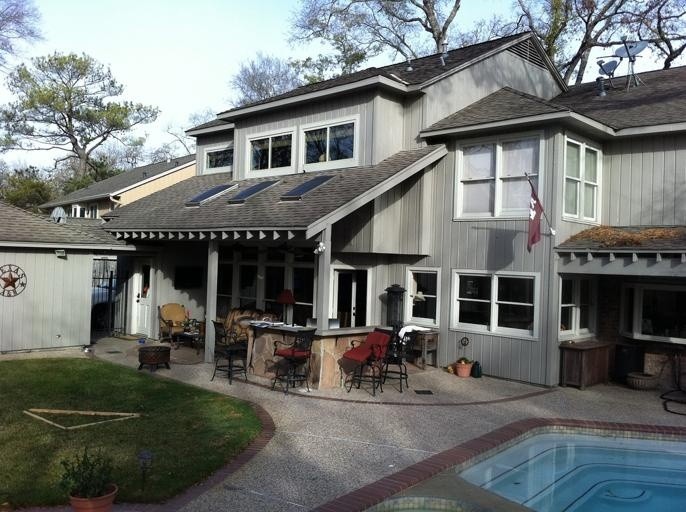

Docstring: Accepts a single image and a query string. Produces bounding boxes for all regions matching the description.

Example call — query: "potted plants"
[457,358,474,377]
[59,445,118,511]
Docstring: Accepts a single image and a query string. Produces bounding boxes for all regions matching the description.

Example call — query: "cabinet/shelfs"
[559,340,610,389]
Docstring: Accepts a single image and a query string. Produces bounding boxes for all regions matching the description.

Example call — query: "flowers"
[180,318,194,329]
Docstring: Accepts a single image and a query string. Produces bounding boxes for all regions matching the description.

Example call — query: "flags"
[525,190,544,254]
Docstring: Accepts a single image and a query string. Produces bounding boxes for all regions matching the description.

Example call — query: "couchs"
[223,308,278,358]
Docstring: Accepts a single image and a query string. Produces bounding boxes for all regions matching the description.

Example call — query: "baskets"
[627,370,658,390]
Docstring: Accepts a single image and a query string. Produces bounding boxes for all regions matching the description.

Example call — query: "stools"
[138,346,171,373]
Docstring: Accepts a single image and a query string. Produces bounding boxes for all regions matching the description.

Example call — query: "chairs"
[158,303,197,350]
[342,328,411,397]
[271,327,317,395]
[209,319,248,385]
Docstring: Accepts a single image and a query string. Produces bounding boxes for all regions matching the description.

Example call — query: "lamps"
[276,290,296,324]
